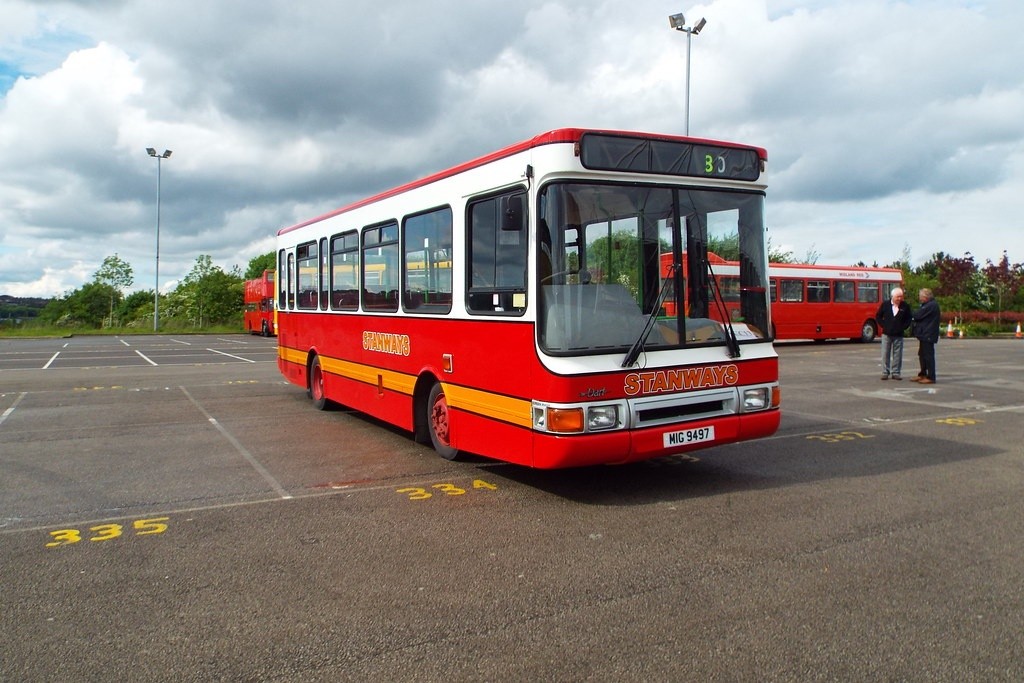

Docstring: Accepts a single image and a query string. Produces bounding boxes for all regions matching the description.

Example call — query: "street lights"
[146,147,173,332]
[667,12,707,140]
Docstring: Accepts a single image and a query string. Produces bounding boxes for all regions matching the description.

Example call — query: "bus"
[275,130,782,469]
[660,251,904,343]
[244,268,277,338]
[273,259,452,336]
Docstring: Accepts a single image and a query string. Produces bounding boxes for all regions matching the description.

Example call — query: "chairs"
[298,288,424,311]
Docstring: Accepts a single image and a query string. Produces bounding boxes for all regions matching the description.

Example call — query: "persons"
[912,288,941,384]
[876,287,912,380]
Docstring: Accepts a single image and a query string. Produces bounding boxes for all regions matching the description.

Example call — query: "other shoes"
[891,374,902,380]
[918,377,935,383]
[880,374,888,380]
[910,376,925,381]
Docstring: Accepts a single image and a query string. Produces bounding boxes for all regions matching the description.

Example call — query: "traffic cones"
[958,328,965,339]
[945,319,956,339]
[1014,320,1024,338]
